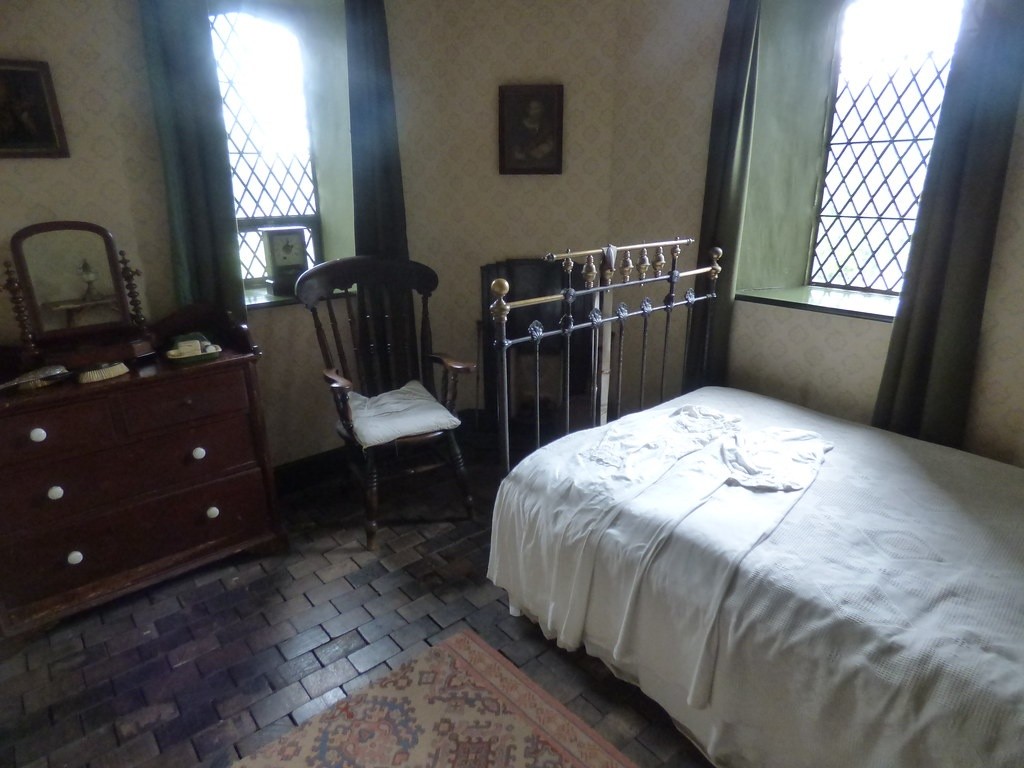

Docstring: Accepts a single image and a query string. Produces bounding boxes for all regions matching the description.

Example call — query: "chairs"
[294,256,474,551]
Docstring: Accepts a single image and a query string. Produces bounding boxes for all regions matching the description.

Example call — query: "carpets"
[228,628,639,768]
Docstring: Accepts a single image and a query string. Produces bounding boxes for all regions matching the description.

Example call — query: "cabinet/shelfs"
[0,309,290,642]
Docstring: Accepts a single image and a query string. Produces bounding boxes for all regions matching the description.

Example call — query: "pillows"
[349,379,462,450]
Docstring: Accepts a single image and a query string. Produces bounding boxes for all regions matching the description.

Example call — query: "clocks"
[260,227,308,296]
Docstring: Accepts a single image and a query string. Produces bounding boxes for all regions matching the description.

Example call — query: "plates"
[166,345,223,366]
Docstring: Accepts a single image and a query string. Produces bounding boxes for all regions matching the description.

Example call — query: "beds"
[488,236,1023,768]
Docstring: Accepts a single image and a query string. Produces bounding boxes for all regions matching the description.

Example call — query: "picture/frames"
[0,56,71,159]
[498,83,563,175]
